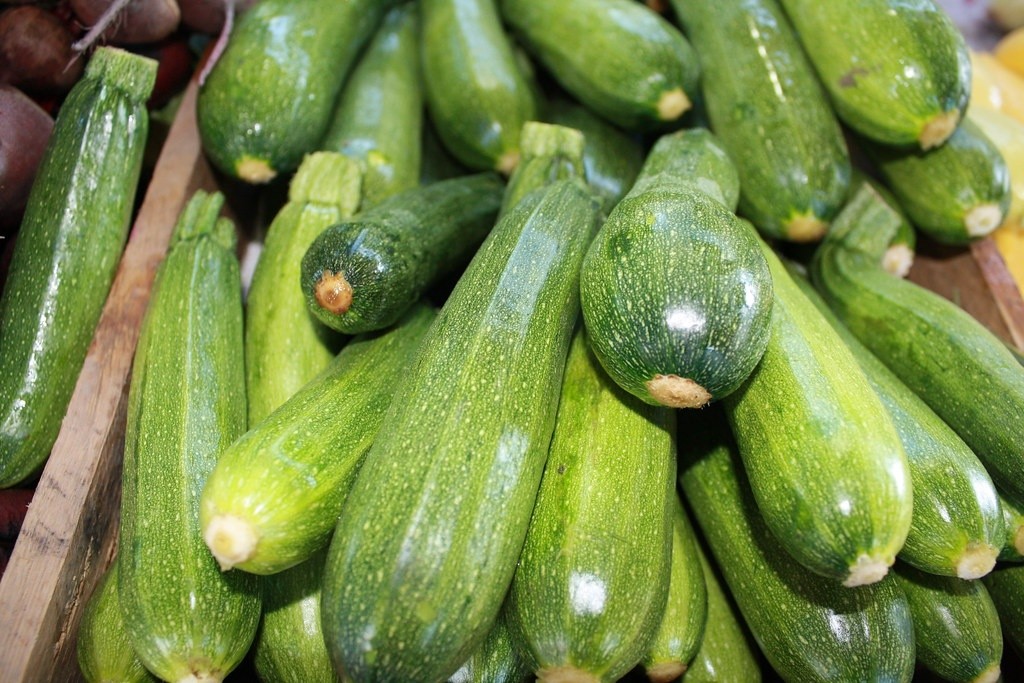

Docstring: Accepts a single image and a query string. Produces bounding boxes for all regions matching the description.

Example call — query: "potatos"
[0,0,252,227]
